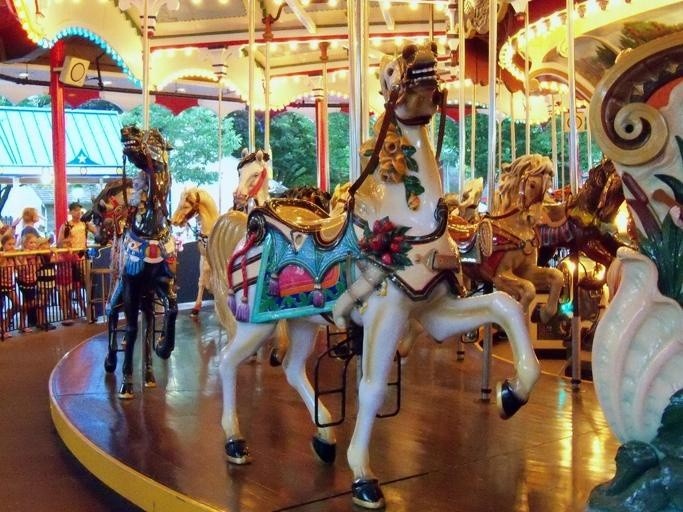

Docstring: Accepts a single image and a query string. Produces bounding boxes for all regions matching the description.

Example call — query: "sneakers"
[0,321,57,338]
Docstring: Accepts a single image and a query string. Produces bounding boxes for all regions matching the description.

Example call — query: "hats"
[70,203,83,210]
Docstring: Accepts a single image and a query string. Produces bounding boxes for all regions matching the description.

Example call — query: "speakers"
[59,56,90,88]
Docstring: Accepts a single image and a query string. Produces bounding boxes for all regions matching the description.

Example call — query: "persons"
[0,234,22,340]
[35,238,57,330]
[0,215,22,242]
[54,236,82,321]
[16,234,44,332]
[57,202,97,318]
[20,208,54,247]
